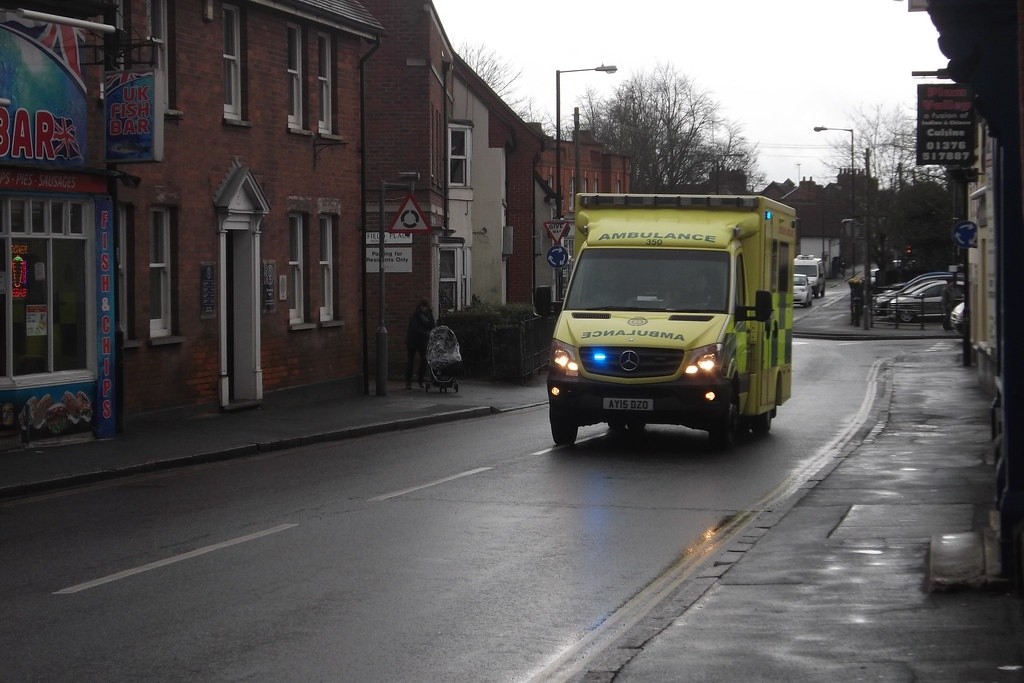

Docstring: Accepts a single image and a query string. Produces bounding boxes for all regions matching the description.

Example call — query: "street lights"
[556,65,617,299]
[814,127,855,278]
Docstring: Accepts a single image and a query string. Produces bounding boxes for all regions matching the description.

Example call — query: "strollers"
[423,326,463,393]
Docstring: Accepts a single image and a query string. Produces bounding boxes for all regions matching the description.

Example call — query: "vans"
[793,254,826,298]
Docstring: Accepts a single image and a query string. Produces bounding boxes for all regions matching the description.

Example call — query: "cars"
[793,273,812,307]
[871,268,967,323]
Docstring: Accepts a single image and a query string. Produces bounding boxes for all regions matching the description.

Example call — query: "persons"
[679,268,729,313]
[941,275,959,331]
[405,296,436,390]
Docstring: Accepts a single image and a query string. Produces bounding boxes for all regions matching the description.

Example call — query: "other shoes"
[417,379,425,387]
[406,383,411,389]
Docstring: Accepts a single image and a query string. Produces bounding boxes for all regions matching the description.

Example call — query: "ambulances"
[547,193,795,444]
